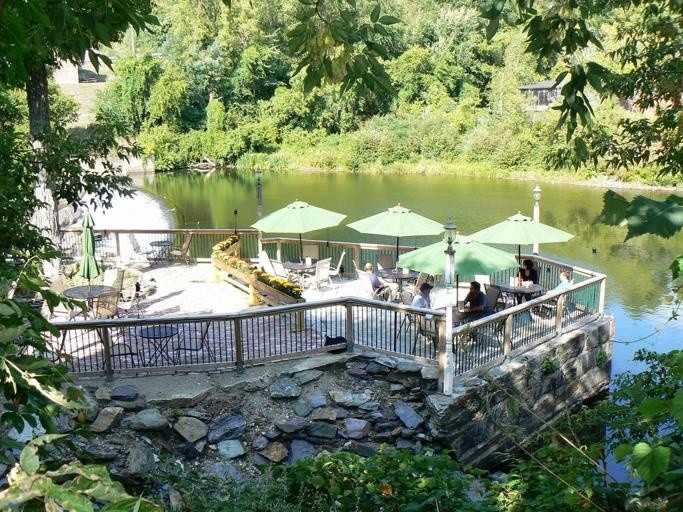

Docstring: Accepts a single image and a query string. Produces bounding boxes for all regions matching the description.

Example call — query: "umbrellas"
[345,202,444,264]
[395,231,518,305]
[466,212,575,269]
[250,197,348,262]
[77,206,101,289]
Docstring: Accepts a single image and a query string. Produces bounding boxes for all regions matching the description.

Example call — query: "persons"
[408,283,433,321]
[544,271,570,292]
[456,281,492,341]
[365,263,391,301]
[517,260,537,305]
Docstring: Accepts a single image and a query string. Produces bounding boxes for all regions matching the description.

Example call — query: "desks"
[139,326,177,367]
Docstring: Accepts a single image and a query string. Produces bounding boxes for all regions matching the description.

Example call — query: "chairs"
[97,328,144,369]
[259,250,346,294]
[176,310,213,365]
[353,255,541,357]
[127,233,197,269]
[48,269,139,321]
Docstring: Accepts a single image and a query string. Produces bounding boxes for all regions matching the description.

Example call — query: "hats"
[419,283,434,292]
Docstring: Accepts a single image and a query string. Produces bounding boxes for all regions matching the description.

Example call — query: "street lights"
[254,165,264,258]
[531,182,542,254]
[439,217,458,396]
